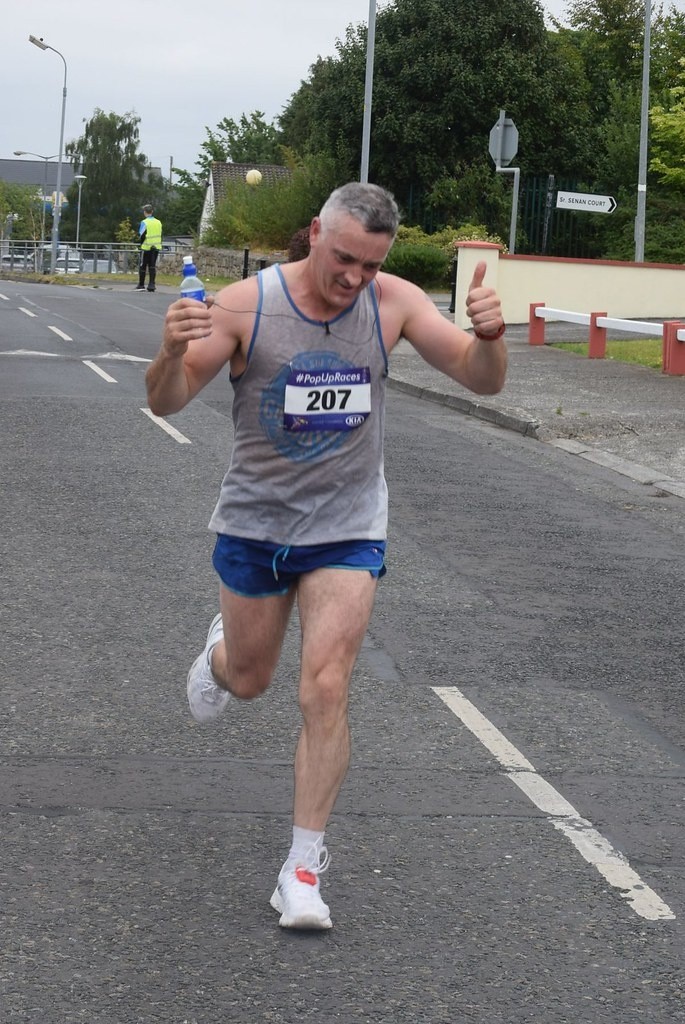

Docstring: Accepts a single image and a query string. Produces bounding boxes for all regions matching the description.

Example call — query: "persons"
[135,205,162,292]
[144,183,507,930]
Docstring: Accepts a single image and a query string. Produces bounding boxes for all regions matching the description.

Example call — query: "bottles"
[179,256,209,339]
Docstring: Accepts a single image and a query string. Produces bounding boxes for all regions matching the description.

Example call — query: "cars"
[30,245,83,271]
[81,259,117,275]
[1,255,34,272]
[54,257,84,273]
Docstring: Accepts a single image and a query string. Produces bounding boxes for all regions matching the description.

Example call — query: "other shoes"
[148,288,155,292]
[132,285,146,291]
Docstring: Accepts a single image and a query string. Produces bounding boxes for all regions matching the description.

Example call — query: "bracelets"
[474,323,505,340]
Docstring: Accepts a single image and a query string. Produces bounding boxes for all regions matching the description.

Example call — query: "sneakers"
[187,610,232,725]
[270,834,332,930]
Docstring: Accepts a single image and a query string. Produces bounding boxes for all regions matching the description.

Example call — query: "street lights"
[13,151,79,273]
[75,175,87,248]
[29,35,67,275]
